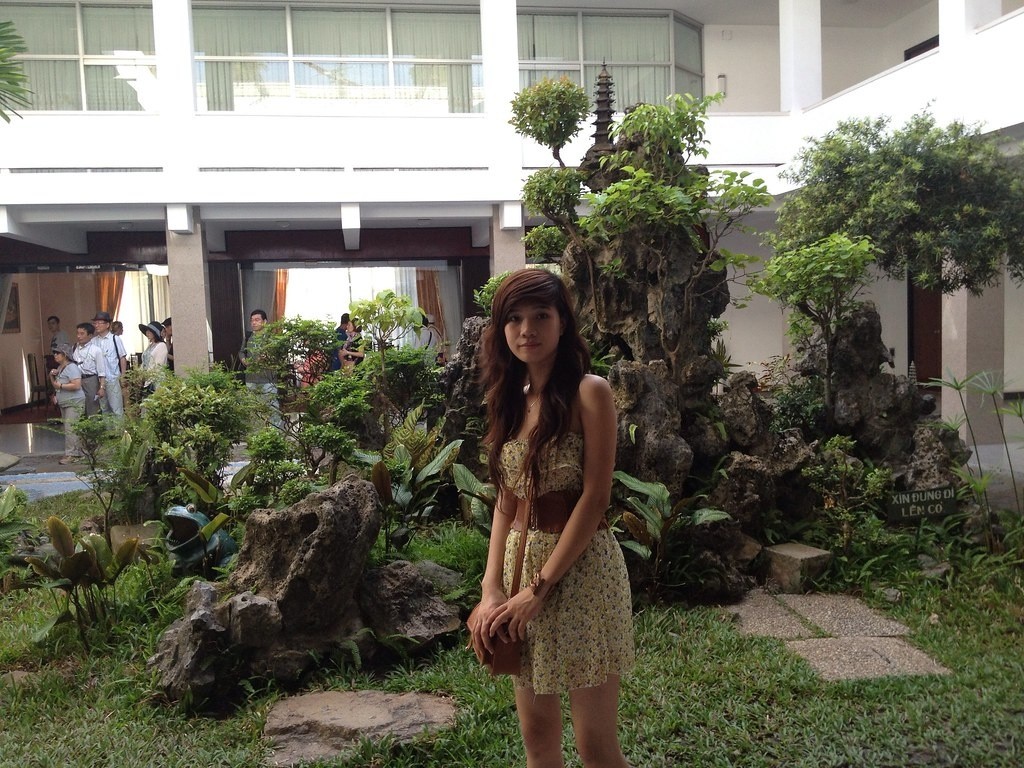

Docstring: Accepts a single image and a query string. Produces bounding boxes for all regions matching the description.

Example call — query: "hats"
[51,343,79,367]
[91,312,113,322]
[426,313,435,323]
[138,321,167,344]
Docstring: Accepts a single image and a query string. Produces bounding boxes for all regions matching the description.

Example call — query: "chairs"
[27,352,48,412]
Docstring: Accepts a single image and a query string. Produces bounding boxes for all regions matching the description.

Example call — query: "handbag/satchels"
[233,330,253,380]
[343,359,354,367]
[51,393,57,406]
[118,361,131,373]
[466,602,522,678]
[46,401,61,425]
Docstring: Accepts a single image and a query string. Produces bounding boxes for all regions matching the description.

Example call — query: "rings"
[51,371,53,372]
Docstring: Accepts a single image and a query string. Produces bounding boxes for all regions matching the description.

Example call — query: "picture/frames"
[2,283,21,334]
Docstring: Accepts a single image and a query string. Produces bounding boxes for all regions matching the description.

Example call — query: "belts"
[513,487,610,534]
[81,373,95,378]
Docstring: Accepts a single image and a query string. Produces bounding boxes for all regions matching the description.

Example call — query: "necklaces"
[527,396,540,413]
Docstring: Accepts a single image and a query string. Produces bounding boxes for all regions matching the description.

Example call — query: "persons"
[333,313,365,371]
[239,309,282,430]
[470,268,632,768]
[46,311,174,465]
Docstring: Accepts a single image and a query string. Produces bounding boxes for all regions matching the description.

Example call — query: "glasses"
[249,318,262,322]
[93,321,107,324]
[53,352,61,355]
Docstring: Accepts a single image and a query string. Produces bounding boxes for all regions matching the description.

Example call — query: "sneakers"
[58,456,77,464]
[78,455,86,461]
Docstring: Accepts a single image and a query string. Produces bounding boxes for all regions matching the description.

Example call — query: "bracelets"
[60,383,62,389]
[530,573,557,600]
[100,386,106,390]
